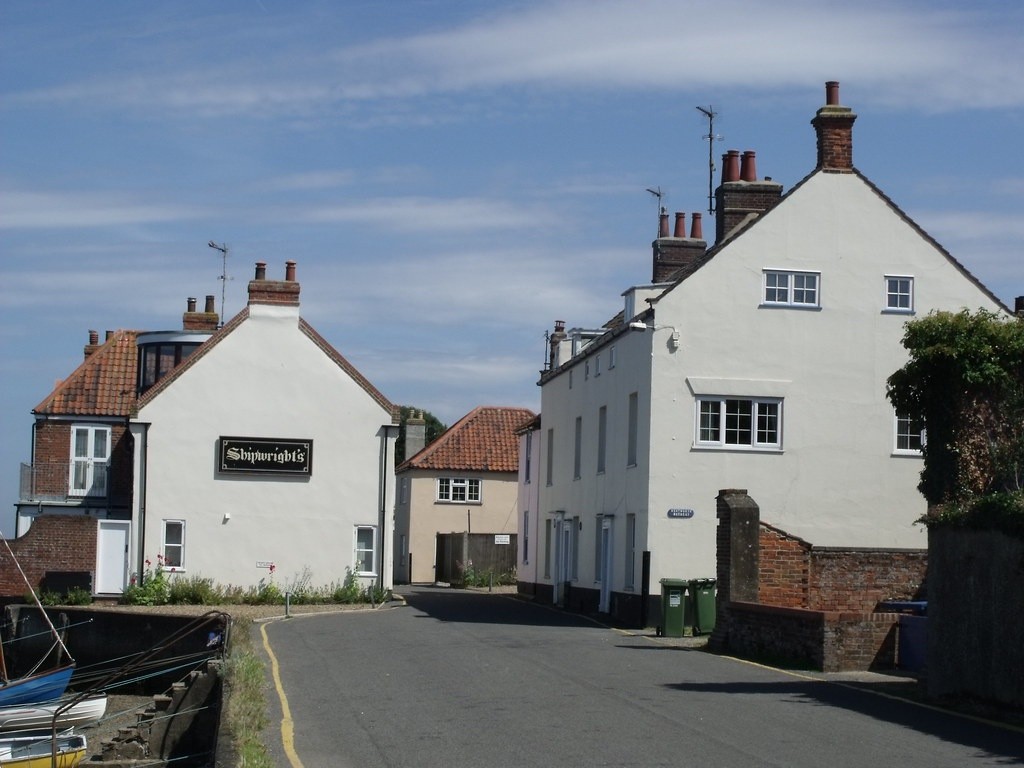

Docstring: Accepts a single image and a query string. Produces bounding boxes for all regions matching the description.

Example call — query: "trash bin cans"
[656,578,689,637]
[687,578,717,636]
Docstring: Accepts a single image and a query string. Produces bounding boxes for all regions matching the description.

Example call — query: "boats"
[0,733,87,768]
[0,725,74,736]
[1,530,76,705]
[0,691,108,730]
